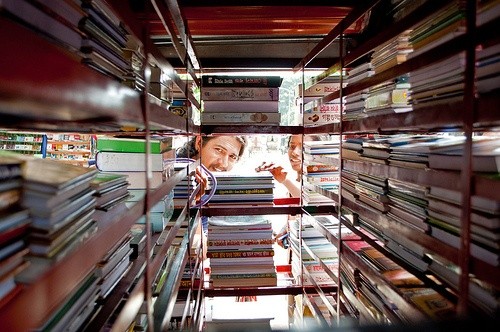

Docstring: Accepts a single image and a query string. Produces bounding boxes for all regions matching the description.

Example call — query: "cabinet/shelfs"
[0,1,500,331]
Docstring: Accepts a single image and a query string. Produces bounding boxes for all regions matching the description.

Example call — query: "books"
[203,174,275,206]
[200,72,284,125]
[289,0,499,331]
[174,173,341,332]
[0,0,204,332]
[208,215,277,287]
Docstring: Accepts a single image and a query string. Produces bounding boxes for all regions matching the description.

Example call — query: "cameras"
[279,234,289,250]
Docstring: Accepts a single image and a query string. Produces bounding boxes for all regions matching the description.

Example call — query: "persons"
[255,134,332,332]
[176,133,257,302]
